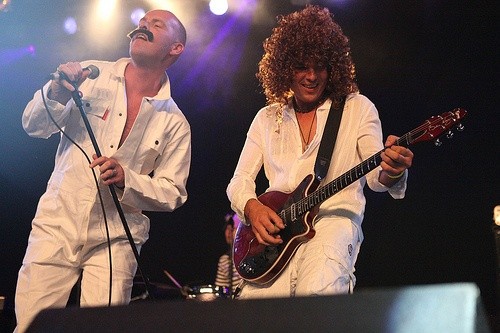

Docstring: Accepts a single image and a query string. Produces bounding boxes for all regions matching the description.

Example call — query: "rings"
[112,169,116,176]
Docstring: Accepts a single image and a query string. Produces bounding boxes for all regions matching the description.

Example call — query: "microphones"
[47,64,100,80]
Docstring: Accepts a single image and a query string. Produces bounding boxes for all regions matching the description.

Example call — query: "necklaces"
[293,96,317,151]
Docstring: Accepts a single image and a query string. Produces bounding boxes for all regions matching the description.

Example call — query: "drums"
[191,284,228,303]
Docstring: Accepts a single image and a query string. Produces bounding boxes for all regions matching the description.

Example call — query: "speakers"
[24,281,492,333]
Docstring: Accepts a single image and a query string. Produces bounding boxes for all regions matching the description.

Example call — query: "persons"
[226,5,412,298]
[215,216,241,293]
[13,9,192,333]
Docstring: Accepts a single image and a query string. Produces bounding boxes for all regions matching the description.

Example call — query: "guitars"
[233,108,467,289]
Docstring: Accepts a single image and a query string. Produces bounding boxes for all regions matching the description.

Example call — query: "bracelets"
[385,171,404,178]
